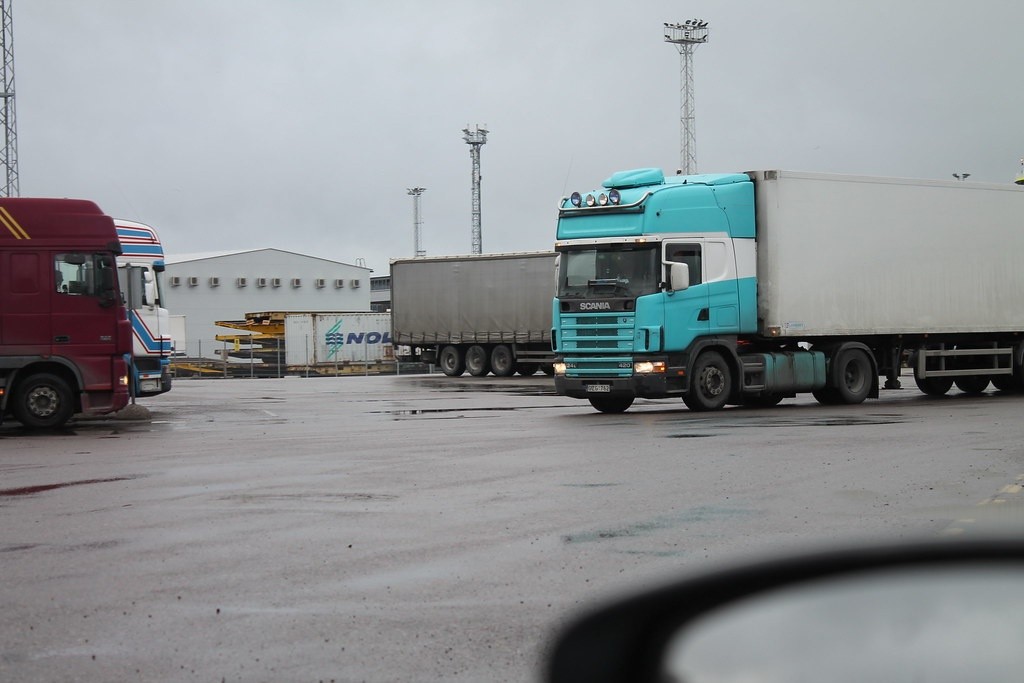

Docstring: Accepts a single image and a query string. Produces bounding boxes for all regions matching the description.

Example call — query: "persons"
[55,271,64,293]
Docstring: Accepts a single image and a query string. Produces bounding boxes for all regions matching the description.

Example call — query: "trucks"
[0,199,136,430]
[112,218,171,398]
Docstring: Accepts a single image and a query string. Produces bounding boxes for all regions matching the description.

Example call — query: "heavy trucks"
[391,255,560,375]
[546,173,1024,410]
